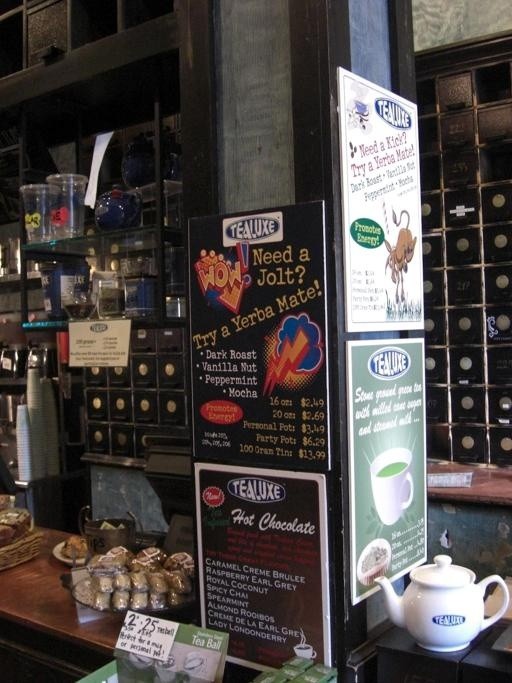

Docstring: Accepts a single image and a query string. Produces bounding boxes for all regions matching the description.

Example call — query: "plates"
[53,539,89,565]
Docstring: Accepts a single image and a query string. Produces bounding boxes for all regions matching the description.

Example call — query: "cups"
[46,173,89,240]
[294,644,317,660]
[19,183,61,245]
[370,447,415,525]
[155,656,176,683]
[16,369,60,481]
[63,288,97,321]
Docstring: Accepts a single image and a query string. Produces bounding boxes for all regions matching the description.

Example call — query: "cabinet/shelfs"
[0,0,220,459]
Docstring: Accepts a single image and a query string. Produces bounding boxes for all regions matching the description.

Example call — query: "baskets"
[0,529,45,570]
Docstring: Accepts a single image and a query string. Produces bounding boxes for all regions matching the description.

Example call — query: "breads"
[62,534,194,612]
[0,494,31,547]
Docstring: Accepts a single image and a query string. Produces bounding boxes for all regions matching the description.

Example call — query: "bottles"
[93,184,143,232]
[124,274,157,321]
[93,272,123,320]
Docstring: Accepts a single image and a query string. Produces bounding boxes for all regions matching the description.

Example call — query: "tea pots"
[373,554,510,654]
